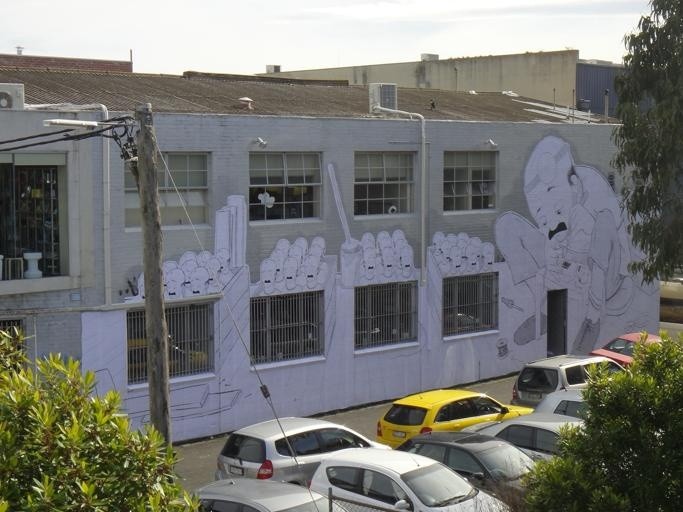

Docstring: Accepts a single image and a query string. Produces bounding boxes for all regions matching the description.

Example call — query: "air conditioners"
[0,83,24,111]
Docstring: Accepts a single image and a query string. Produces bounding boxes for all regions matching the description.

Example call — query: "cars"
[189,477,349,512]
[532,388,592,421]
[461,412,587,461]
[214,416,394,490]
[376,389,535,450]
[394,430,541,507]
[589,332,663,369]
[309,447,511,512]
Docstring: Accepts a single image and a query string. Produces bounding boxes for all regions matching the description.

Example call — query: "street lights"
[43,103,171,456]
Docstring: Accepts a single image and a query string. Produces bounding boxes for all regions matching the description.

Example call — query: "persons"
[492,135,634,357]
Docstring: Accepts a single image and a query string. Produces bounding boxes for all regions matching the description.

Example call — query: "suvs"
[510,354,632,409]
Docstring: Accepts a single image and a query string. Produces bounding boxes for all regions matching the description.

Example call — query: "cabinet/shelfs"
[18,168,61,276]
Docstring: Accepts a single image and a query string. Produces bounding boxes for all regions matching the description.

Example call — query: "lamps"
[483,138,498,148]
[254,136,267,147]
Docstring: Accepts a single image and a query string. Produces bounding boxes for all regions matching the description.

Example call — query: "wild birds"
[428,97,435,112]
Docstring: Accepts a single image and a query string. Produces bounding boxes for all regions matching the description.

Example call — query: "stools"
[5,257,24,280]
